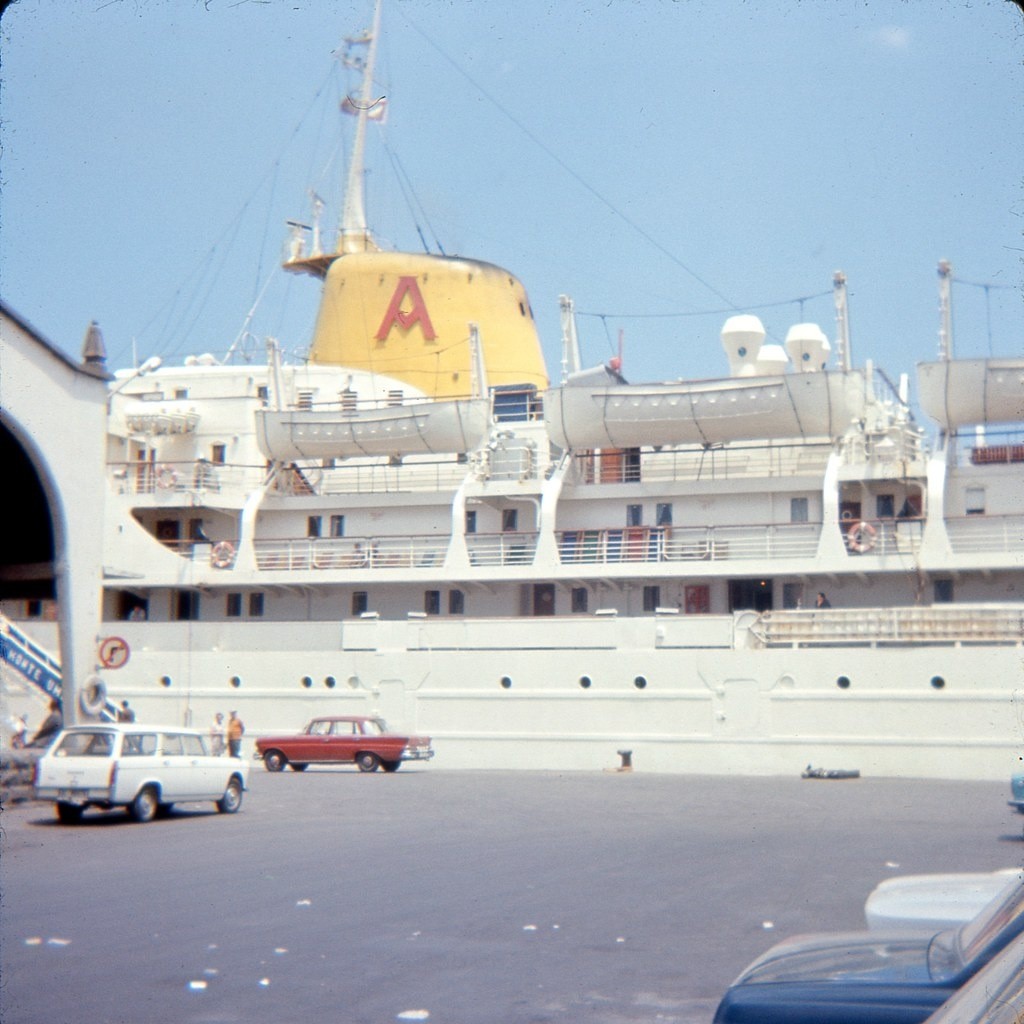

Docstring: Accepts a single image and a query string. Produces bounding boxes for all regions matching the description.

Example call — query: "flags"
[341,98,388,122]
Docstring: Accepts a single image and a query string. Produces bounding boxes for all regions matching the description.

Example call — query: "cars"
[256,715,435,775]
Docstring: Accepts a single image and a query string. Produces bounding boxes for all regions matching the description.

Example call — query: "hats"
[229,709,237,713]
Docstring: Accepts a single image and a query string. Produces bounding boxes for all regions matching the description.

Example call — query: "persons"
[128,605,146,621]
[119,701,135,723]
[815,593,831,609]
[211,709,245,756]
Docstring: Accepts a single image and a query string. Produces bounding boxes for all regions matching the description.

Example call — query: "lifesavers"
[210,540,235,568]
[153,464,178,488]
[849,521,877,553]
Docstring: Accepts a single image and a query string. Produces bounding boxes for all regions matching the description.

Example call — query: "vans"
[31,723,249,825]
